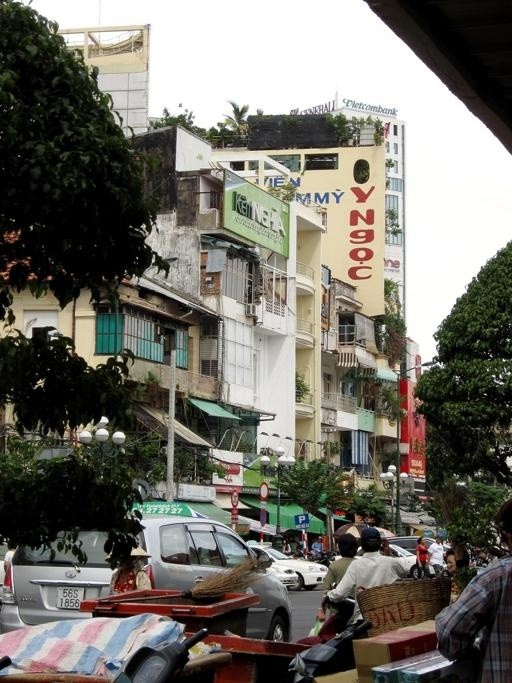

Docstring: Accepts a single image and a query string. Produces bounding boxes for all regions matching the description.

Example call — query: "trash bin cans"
[79,590,260,638]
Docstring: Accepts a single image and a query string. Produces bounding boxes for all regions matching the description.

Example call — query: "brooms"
[98,558,265,615]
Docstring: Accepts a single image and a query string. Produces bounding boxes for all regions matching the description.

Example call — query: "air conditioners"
[247,303,257,316]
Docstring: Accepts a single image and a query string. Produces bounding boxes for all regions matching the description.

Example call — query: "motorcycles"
[307,582,355,641]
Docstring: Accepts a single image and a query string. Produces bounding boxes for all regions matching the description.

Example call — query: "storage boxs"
[305,619,458,683]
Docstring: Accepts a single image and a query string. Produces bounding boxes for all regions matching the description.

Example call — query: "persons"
[424,534,445,578]
[315,532,359,622]
[311,536,324,552]
[321,526,411,627]
[281,537,292,553]
[435,499,512,683]
[415,536,427,578]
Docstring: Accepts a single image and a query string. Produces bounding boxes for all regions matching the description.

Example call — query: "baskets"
[355,569,452,639]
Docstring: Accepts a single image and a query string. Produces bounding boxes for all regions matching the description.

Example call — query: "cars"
[248,544,329,591]
[330,536,476,580]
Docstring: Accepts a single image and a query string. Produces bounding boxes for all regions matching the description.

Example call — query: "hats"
[361,527,382,542]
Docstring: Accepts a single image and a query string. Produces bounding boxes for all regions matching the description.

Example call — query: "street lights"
[380,465,408,533]
[260,447,296,545]
[78,414,127,466]
[392,356,438,536]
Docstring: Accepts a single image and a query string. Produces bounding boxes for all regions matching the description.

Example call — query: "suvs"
[0,514,293,642]
